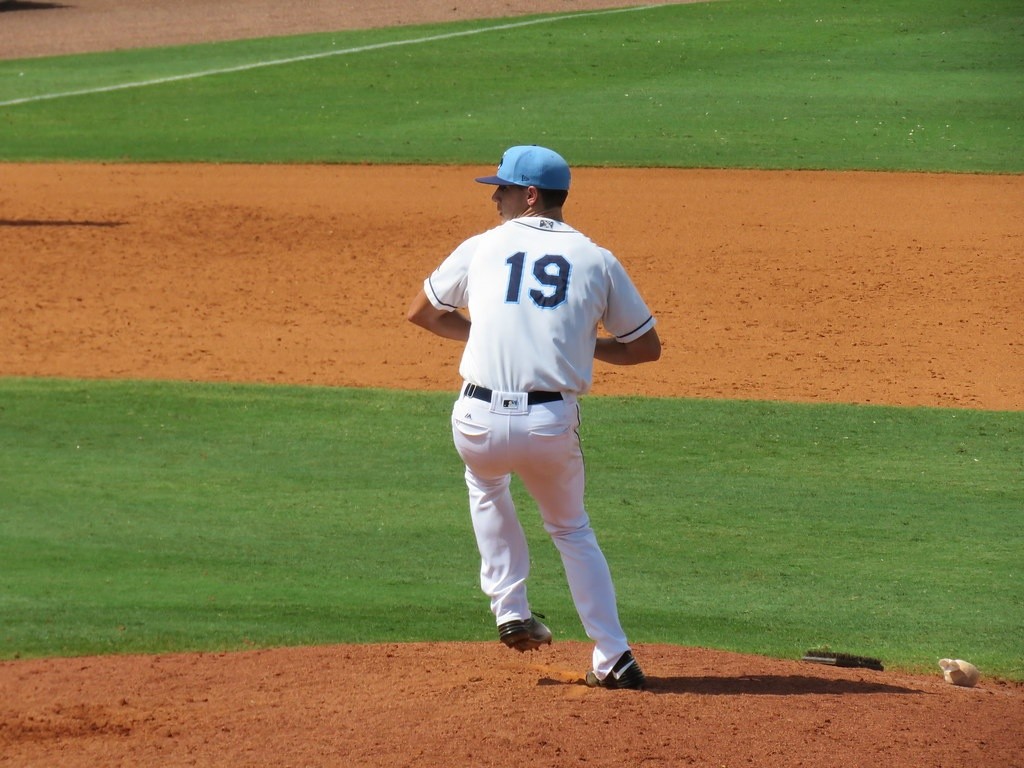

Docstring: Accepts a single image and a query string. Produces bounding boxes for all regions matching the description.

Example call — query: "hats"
[474,145,571,191]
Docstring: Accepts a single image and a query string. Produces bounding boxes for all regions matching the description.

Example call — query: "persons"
[405,143,661,689]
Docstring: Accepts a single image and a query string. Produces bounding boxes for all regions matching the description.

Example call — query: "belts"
[463,383,563,406]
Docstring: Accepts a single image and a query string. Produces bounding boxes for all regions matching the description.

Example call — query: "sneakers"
[498,612,552,653]
[585,652,645,690]
